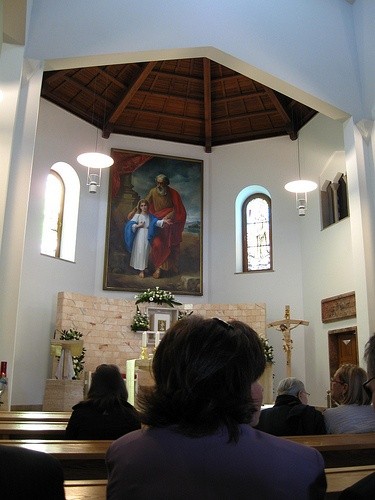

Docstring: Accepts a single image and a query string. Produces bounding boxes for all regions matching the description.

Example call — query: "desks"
[43,379,86,412]
[0,408,375,461]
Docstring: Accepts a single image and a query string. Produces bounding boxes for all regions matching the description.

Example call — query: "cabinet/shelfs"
[126,308,178,407]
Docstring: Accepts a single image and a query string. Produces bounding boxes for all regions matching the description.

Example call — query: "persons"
[330,332,375,500]
[269,320,303,353]
[107,317,328,500]
[252,377,327,436]
[322,363,375,435]
[0,445,65,500]
[64,364,141,440]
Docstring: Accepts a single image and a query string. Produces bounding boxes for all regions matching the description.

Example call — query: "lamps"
[284,138,318,217]
[76,119,115,194]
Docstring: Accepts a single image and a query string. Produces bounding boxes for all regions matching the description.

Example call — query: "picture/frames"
[102,147,205,296]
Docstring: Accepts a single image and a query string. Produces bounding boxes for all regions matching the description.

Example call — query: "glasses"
[301,391,310,397]
[362,375,375,408]
[212,317,261,355]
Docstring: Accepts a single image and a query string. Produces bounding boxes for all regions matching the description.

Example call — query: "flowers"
[56,327,86,340]
[71,349,86,379]
[134,285,183,307]
[130,306,151,331]
[259,333,277,367]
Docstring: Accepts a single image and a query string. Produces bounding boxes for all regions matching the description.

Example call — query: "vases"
[151,302,169,308]
[63,340,84,357]
